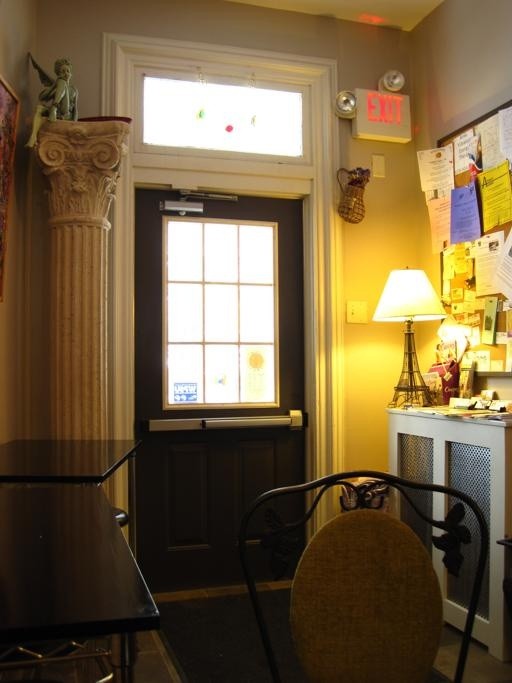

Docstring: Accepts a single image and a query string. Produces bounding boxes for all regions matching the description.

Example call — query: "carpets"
[153,588,454,681]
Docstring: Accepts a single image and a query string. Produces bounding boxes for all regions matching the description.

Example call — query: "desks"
[0,439,142,527]
[0,483,163,682]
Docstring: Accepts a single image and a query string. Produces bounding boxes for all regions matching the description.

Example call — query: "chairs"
[238,471,490,682]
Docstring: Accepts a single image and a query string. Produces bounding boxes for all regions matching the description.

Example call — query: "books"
[417,358,512,423]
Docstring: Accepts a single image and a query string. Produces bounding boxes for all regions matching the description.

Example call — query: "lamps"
[372,266,446,409]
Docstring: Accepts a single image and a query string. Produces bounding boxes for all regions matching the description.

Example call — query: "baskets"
[337,168,365,223]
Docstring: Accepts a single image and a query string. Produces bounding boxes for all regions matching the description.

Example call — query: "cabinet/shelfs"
[384,405,510,662]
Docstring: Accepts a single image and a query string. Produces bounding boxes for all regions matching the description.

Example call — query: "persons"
[435,344,444,361]
[23,50,79,150]
